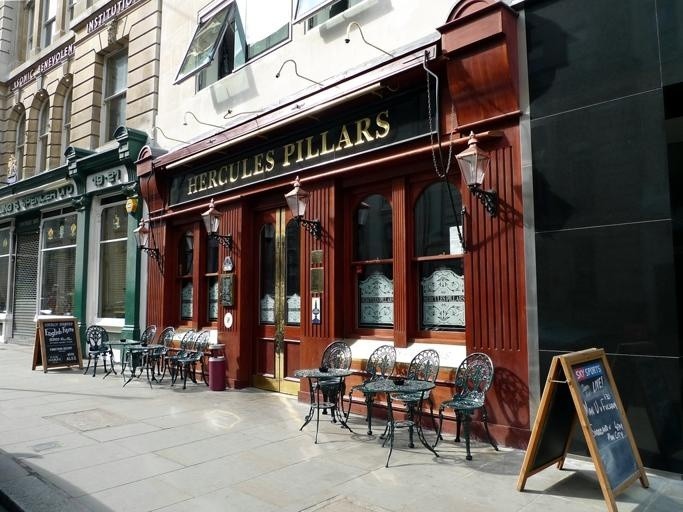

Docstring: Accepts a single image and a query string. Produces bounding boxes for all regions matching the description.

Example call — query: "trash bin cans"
[207,343,226,391]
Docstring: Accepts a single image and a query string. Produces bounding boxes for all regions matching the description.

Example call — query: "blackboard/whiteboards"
[559,347,644,499]
[38,317,82,368]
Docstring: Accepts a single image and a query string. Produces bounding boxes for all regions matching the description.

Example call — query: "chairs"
[320,341,351,423]
[400,349,442,447]
[83,325,209,391]
[432,352,498,460]
[342,346,396,428]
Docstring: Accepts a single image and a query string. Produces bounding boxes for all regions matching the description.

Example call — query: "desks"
[294,367,355,443]
[363,379,440,468]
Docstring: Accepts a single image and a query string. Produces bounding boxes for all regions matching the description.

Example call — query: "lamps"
[2,232,8,249]
[47,227,54,241]
[456,130,498,217]
[201,198,231,251]
[285,176,320,241]
[133,218,159,259]
[112,208,121,232]
[70,213,78,239]
[150,22,394,144]
